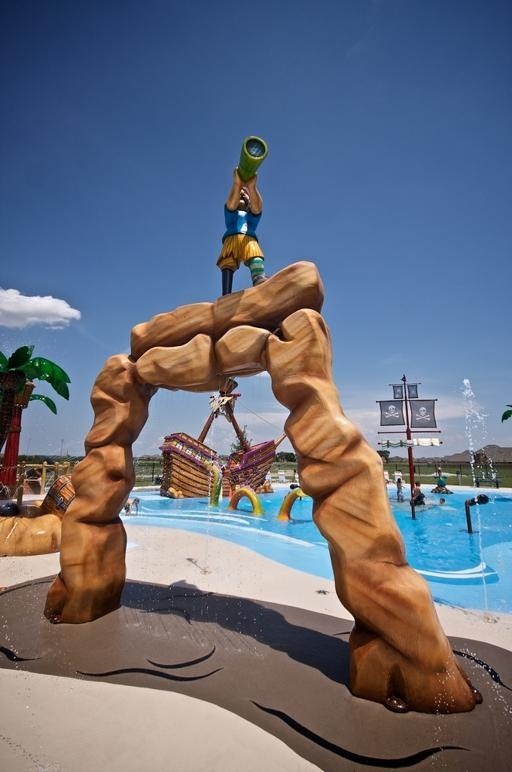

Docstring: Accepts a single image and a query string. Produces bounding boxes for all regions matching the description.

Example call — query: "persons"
[294,460,445,506]
[124,498,139,514]
[216,167,269,297]
